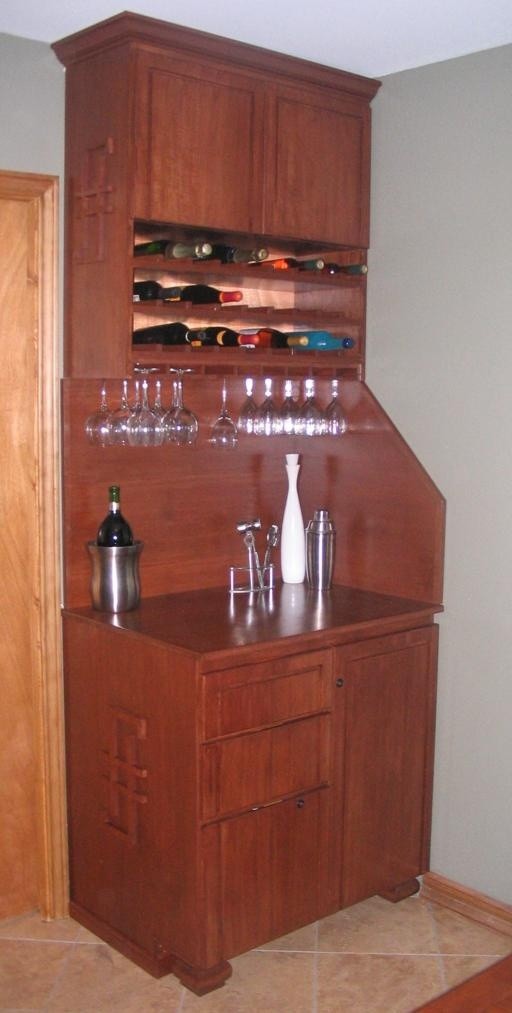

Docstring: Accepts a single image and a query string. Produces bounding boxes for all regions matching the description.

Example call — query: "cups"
[86,542,145,611]
[304,510,333,590]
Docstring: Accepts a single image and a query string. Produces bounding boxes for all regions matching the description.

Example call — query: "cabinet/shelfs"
[137,11,384,248]
[335,606,442,913]
[132,221,367,371]
[60,609,330,994]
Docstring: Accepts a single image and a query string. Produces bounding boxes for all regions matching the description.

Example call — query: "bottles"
[189,327,260,347]
[237,379,259,436]
[97,485,133,546]
[313,263,368,276]
[280,453,306,584]
[249,259,324,271]
[194,244,267,264]
[254,379,283,436]
[134,281,206,301]
[278,379,296,435]
[295,378,326,436]
[133,239,212,261]
[282,331,354,351]
[324,380,349,435]
[181,283,242,304]
[132,322,205,345]
[233,329,308,347]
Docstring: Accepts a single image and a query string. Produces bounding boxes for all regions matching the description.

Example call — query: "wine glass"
[84,365,199,448]
[210,376,239,450]
